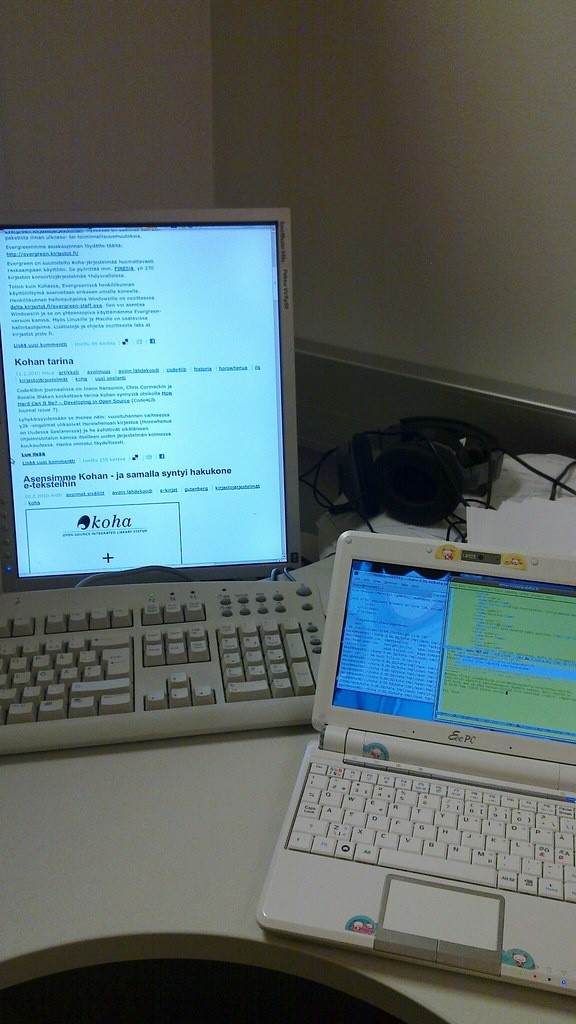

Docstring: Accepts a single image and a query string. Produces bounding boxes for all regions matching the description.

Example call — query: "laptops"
[253,528,576,997]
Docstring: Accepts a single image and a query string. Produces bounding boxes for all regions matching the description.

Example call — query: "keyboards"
[0,581,328,759]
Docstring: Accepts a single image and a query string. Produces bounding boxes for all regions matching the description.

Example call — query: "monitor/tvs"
[0,204,300,585]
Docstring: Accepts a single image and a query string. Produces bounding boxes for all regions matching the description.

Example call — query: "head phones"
[333,415,507,525]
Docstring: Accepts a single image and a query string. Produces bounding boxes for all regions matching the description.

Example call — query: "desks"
[0,532,575,1023]
